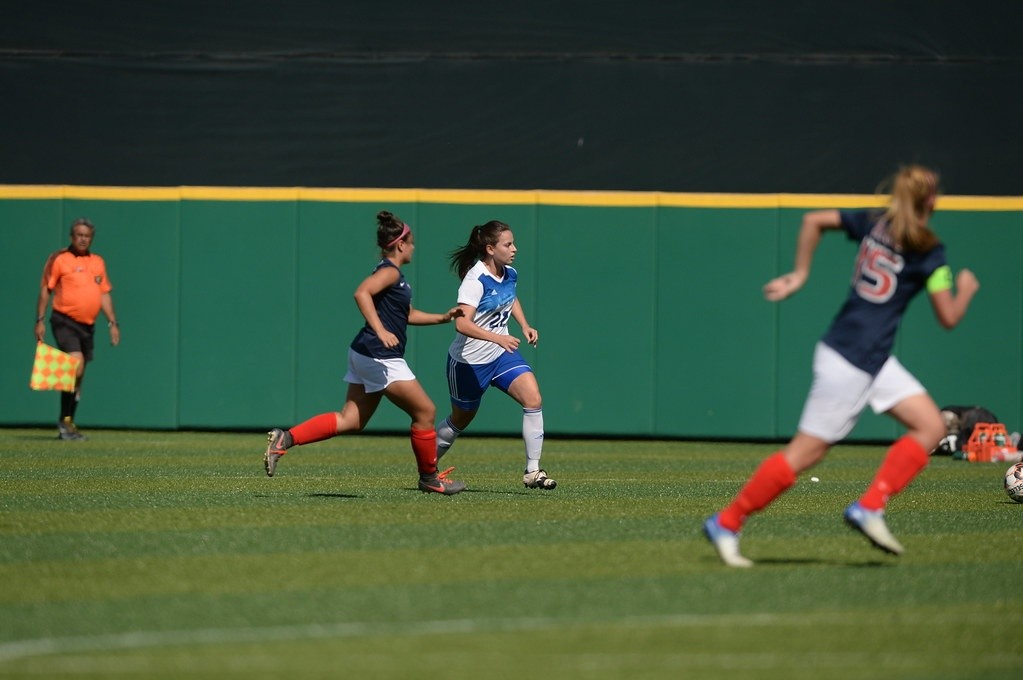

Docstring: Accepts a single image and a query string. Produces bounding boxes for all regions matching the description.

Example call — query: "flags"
[30,342,81,393]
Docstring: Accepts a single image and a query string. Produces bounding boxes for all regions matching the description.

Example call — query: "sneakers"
[522,468,557,490]
[844,502,905,555]
[418,467,466,496]
[704,515,753,568]
[263,428,287,477]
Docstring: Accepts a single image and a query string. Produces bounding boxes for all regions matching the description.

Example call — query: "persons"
[436,220,558,491]
[34,217,120,439]
[699,162,980,569]
[263,211,466,495]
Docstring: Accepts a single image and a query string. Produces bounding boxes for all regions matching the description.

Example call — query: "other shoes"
[59,421,89,442]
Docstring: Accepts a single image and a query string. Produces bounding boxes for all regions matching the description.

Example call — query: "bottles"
[980,431,1006,446]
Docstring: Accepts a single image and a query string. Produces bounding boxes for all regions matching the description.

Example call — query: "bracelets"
[109,320,118,327]
[36,315,45,323]
